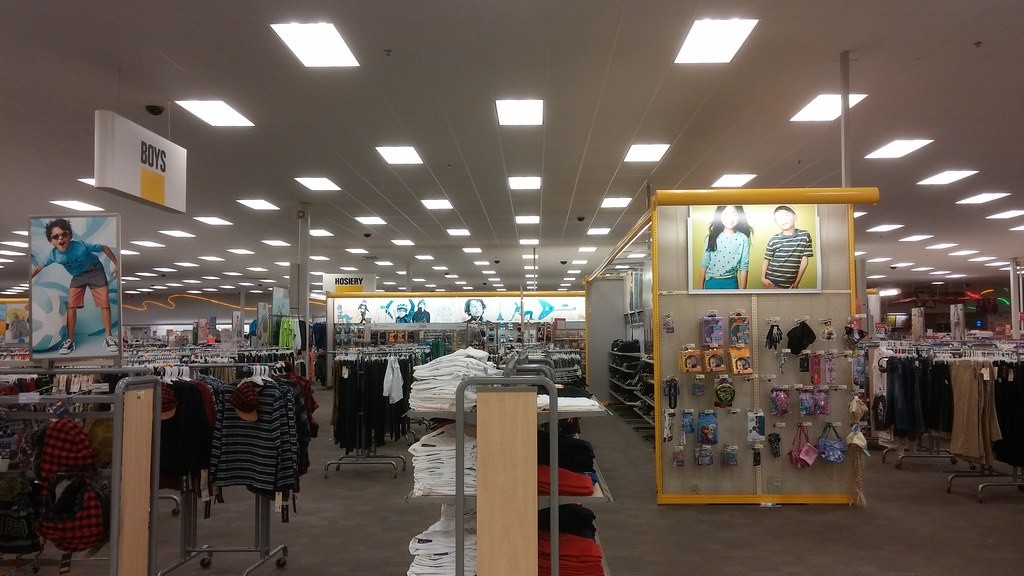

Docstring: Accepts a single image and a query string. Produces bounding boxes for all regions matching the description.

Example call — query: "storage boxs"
[679,316,754,376]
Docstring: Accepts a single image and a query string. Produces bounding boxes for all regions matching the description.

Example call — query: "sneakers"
[57,339,75,354]
[105,335,119,352]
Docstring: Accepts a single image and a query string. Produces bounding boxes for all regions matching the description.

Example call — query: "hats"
[230,383,258,421]
[846,432,871,457]
[161,384,176,419]
[773,204,796,214]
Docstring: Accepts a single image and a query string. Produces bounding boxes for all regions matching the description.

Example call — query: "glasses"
[49,231,69,240]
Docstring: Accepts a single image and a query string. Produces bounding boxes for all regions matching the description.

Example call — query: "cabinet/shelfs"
[407,351,655,576]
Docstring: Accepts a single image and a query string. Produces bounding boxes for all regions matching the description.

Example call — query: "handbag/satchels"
[789,427,819,468]
[45,477,81,521]
[816,423,846,464]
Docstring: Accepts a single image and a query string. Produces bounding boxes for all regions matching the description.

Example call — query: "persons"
[710,355,722,367]
[736,359,749,369]
[760,205,814,289]
[32,218,118,354]
[462,299,493,323]
[686,356,697,368]
[699,206,754,290]
[337,299,430,324]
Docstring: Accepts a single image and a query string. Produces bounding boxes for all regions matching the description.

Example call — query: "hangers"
[500,341,582,361]
[875,337,1024,364]
[0,313,432,386]
